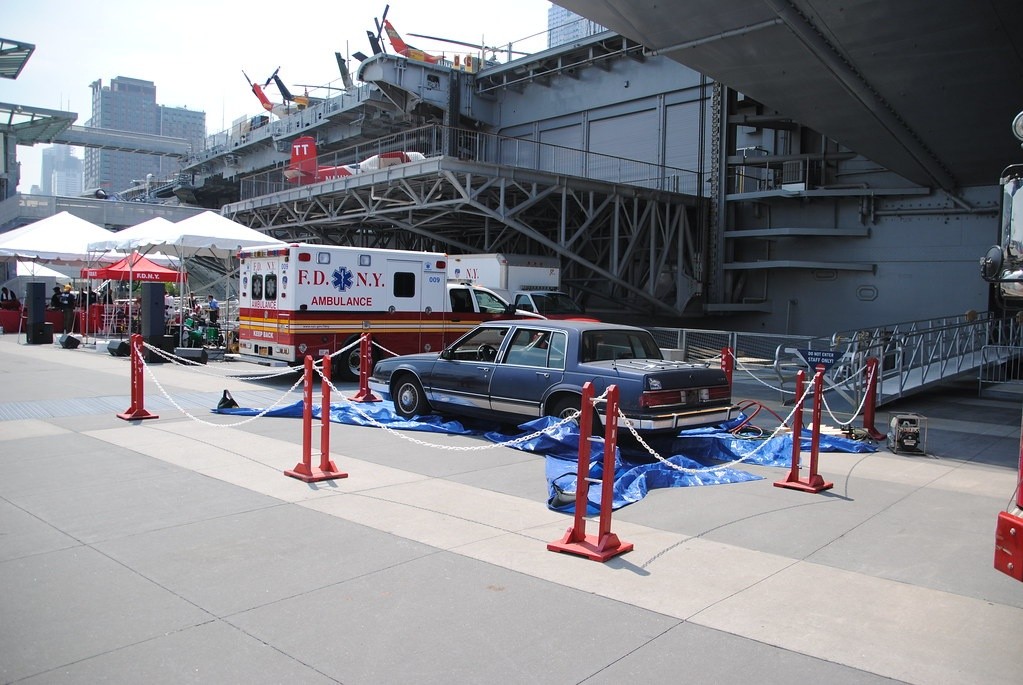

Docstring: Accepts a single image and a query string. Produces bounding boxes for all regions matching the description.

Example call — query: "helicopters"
[242,3,533,118]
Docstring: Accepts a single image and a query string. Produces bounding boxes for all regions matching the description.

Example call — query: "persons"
[165,292,173,309]
[51,287,62,307]
[77,287,87,306]
[205,295,219,323]
[86,286,99,305]
[1,287,16,301]
[59,284,75,334]
[188,291,197,308]
[103,287,113,305]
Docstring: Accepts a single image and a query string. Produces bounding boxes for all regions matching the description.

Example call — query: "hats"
[63,285,72,292]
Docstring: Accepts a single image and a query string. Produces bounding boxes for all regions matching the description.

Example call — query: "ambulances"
[232,243,549,380]
[444,253,585,331]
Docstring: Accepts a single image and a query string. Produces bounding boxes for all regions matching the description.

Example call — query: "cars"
[368,320,731,442]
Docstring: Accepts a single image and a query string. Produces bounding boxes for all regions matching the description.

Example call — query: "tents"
[80,251,187,333]
[128,212,287,346]
[0,210,185,285]
[84,217,231,353]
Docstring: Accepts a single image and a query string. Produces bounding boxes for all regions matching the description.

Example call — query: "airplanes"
[282,136,427,185]
